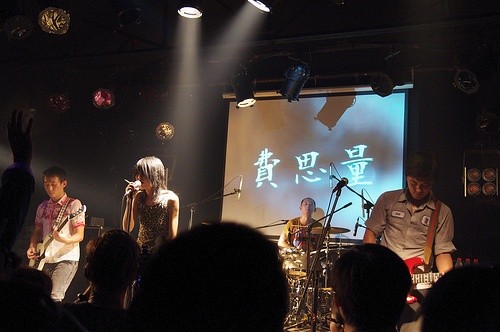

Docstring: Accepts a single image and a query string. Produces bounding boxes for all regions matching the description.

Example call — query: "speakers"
[62,227,101,301]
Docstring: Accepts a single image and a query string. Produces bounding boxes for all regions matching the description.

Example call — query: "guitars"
[403,257,443,306]
[30,205,86,269]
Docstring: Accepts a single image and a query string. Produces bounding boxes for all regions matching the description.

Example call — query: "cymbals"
[311,225,350,235]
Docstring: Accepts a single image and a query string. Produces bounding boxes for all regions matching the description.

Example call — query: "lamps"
[88,63,130,113]
[369,48,404,97]
[231,72,257,109]
[275,56,311,103]
[452,39,496,97]
[48,78,73,113]
[248,0,274,15]
[175,0,203,19]
[0,0,34,40]
[462,149,500,201]
[37,0,72,36]
[111,0,145,33]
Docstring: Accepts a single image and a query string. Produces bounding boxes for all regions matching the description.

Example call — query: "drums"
[281,252,310,280]
[308,248,349,289]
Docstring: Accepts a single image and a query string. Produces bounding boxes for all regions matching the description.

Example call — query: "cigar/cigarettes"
[124,179,130,184]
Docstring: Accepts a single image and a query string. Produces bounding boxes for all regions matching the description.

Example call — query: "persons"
[0,220,290,332]
[27,165,85,301]
[362,159,457,332]
[122,157,179,311]
[278,197,323,253]
[331,243,500,332]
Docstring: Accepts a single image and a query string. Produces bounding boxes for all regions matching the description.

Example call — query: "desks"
[85,225,110,237]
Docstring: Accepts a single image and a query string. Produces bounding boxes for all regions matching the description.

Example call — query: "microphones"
[333,178,349,192]
[329,164,333,187]
[124,179,141,195]
[236,177,243,200]
[362,190,365,217]
[353,218,359,235]
